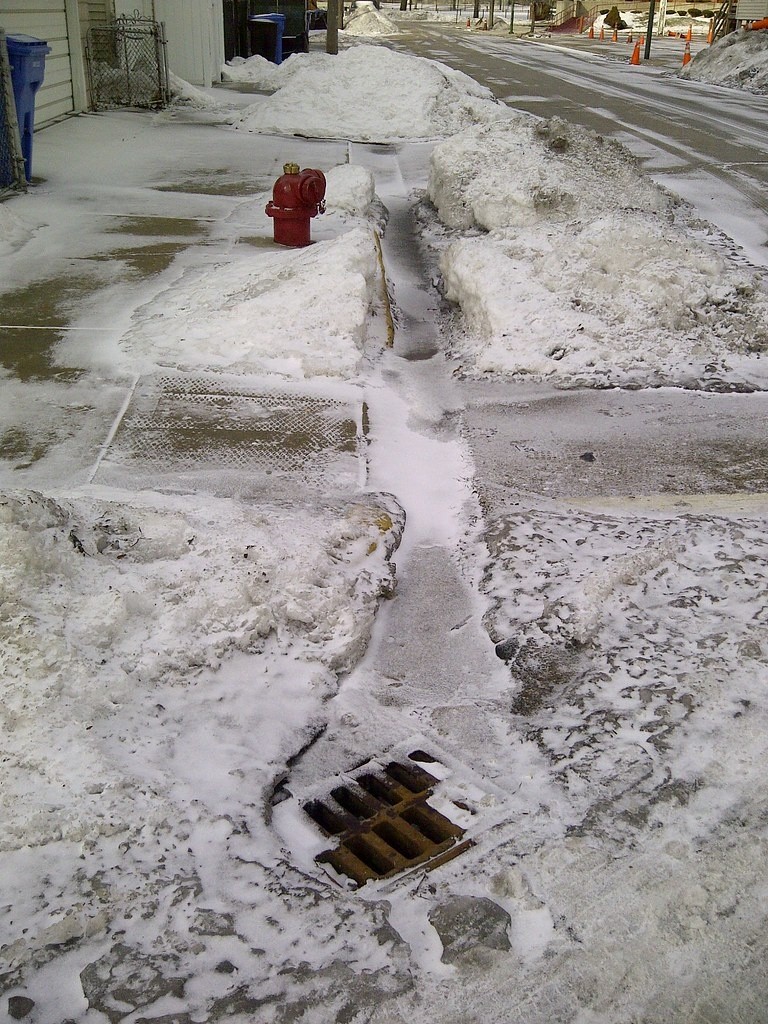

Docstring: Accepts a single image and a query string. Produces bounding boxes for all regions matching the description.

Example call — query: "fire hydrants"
[264,163,327,250]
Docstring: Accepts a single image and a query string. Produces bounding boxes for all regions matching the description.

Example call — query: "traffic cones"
[611,29,617,42]
[667,31,686,38]
[685,24,693,42]
[467,18,470,28]
[707,29,712,43]
[639,36,644,46]
[483,19,488,30]
[682,42,691,65]
[588,28,594,40]
[599,28,605,41]
[743,17,768,31]
[629,41,641,65]
[626,36,633,43]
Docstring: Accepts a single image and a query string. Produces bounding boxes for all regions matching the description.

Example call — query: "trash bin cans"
[248,19,278,63]
[248,12,286,65]
[6,33,52,182]
